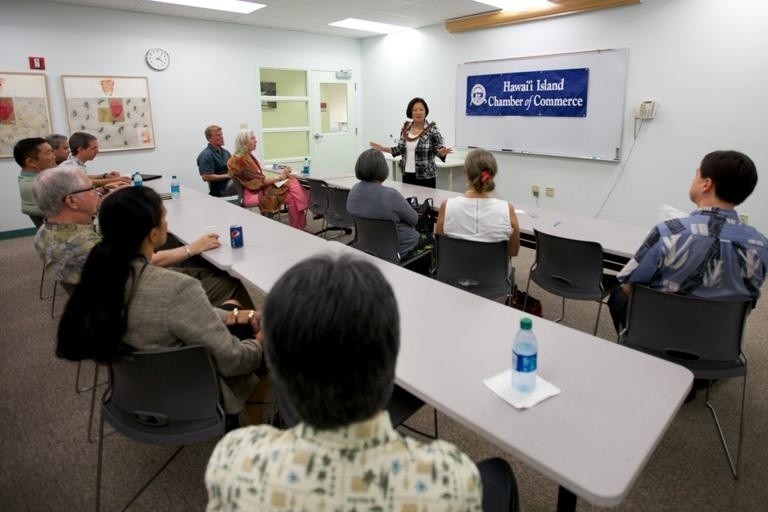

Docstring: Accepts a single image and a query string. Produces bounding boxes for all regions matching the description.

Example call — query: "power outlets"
[532,185,540,195]
[545,188,554,197]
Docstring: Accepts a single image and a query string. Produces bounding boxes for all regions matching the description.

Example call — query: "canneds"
[230,225,243,248]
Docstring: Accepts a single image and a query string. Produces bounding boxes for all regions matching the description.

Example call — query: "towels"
[482,368,562,413]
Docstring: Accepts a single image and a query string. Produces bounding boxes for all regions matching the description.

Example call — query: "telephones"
[633,100,656,140]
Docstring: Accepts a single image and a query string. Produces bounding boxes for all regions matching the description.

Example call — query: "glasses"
[63,181,97,203]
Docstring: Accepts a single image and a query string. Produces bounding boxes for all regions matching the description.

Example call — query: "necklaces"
[412,121,423,135]
[466,191,489,195]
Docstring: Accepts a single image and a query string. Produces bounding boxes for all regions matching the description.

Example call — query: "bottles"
[171,176,180,200]
[511,319,538,393]
[134,172,142,186]
[303,158,310,175]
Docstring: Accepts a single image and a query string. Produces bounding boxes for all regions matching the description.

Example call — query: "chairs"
[347,215,430,276]
[616,283,752,481]
[231,176,243,207]
[96,344,227,512]
[524,227,620,335]
[323,186,350,243]
[305,178,327,234]
[431,232,515,308]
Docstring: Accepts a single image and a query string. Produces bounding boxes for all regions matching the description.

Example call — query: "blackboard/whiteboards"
[451,58,642,159]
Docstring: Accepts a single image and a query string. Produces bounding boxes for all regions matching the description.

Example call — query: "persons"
[435,149,520,257]
[197,126,239,197]
[368,98,454,189]
[226,131,309,231]
[347,147,435,276]
[47,135,135,184]
[55,132,132,186]
[608,151,768,405]
[52,186,269,416]
[13,138,129,219]
[205,256,519,512]
[34,168,257,311]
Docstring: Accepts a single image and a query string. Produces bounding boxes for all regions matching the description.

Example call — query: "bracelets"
[247,309,254,326]
[233,307,238,325]
[102,185,108,192]
[184,245,192,259]
[101,172,107,178]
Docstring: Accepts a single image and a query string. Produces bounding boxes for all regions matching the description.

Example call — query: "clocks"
[145,48,170,71]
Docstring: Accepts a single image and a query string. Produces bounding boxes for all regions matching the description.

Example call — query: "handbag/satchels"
[405,197,435,235]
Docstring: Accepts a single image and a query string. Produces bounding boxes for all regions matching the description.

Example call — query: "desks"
[263,160,448,214]
[385,153,467,193]
[110,177,327,271]
[229,240,694,511]
[432,193,647,272]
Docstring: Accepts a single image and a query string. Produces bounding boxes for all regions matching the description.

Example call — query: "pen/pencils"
[556,217,562,228]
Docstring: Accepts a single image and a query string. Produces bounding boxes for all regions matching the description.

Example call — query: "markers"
[468,146,477,149]
[502,148,513,152]
